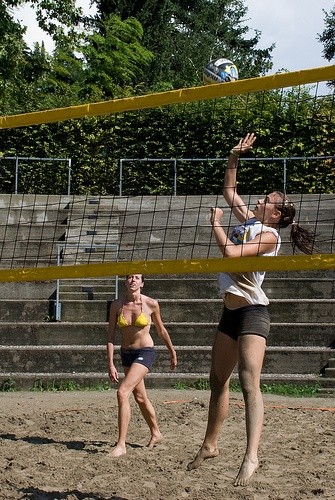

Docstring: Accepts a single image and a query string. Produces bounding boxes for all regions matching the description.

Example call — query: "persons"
[107,273,178,457]
[186,132,321,486]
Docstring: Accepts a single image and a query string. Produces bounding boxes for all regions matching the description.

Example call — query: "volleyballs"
[203,58,238,86]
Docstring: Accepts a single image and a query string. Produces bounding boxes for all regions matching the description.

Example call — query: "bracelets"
[230,151,239,157]
